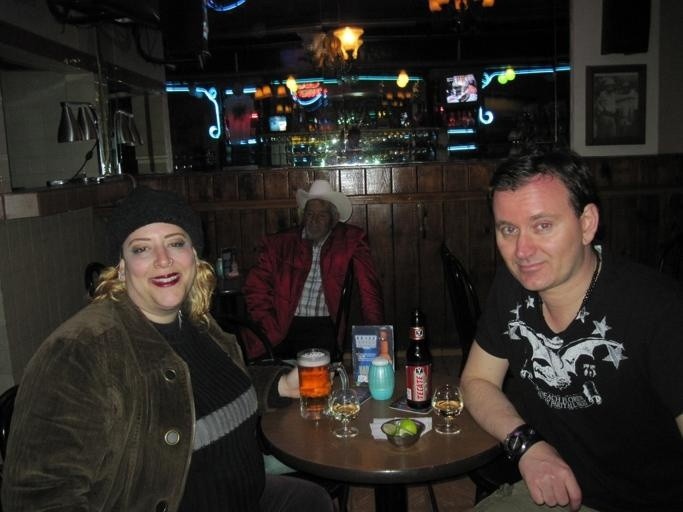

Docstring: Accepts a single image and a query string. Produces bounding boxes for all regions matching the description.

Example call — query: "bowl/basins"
[381,419,425,446]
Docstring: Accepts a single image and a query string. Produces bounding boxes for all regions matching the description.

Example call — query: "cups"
[297,348,347,420]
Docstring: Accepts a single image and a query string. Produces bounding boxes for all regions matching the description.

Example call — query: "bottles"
[405,312,432,408]
[216,258,225,279]
[294,130,441,169]
[368,358,394,400]
[375,327,393,365]
[446,109,475,127]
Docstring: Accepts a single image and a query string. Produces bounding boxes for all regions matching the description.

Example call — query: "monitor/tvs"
[440,70,483,109]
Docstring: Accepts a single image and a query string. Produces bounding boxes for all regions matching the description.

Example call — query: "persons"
[595,78,616,115]
[1,185,334,512]
[458,149,682,511]
[240,179,383,365]
[616,81,638,137]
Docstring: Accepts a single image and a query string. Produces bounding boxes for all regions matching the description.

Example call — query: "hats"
[109,186,204,268]
[296,179,352,223]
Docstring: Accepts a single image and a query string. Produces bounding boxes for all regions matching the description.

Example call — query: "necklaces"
[572,248,600,323]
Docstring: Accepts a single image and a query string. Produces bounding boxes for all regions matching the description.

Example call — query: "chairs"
[0,385,18,461]
[331,263,353,358]
[436,246,481,364]
[210,290,254,366]
[85,262,106,296]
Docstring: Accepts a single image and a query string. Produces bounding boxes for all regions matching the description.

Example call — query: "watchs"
[502,422,547,469]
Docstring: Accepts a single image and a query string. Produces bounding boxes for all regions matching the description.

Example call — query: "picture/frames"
[585,65,646,146]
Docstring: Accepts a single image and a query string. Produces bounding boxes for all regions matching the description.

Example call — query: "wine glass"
[329,390,360,439]
[432,385,463,434]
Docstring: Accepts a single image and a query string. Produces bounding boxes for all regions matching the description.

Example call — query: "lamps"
[333,0,364,60]
